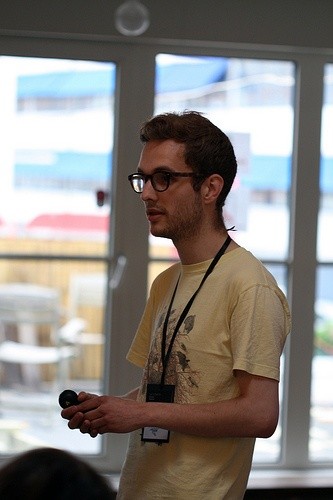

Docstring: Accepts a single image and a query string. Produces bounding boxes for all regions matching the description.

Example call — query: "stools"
[0,284,70,410]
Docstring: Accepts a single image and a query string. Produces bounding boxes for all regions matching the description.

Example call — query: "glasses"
[128,171,191,194]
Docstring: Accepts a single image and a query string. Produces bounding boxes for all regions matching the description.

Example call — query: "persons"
[60,111,292,500]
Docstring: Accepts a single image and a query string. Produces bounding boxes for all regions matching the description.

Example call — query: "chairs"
[59,274,109,347]
